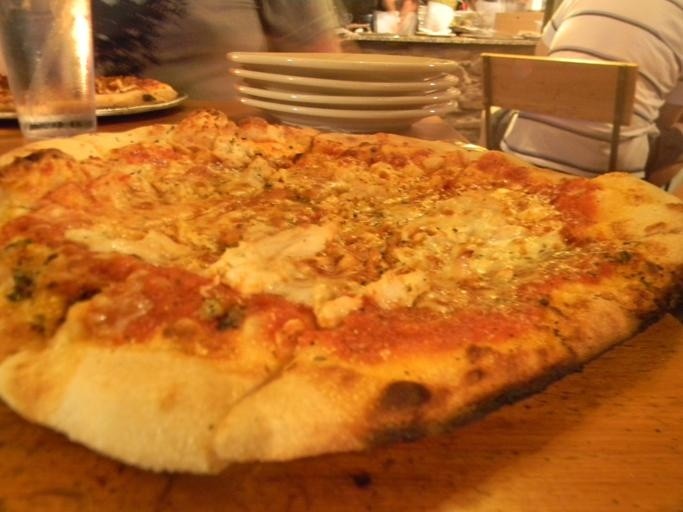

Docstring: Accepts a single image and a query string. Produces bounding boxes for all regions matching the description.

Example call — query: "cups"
[374,9,400,38]
[0,0,101,138]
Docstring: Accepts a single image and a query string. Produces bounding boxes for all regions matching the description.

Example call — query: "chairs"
[478,51,639,173]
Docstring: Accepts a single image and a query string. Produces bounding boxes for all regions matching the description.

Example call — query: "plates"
[227,52,462,134]
[2,91,190,118]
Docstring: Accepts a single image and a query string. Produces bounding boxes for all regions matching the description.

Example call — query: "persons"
[89,0,341,124]
[476,1,682,182]
[372,0,419,34]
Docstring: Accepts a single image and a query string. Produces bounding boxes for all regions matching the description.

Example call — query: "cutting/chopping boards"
[1,310,683,512]
